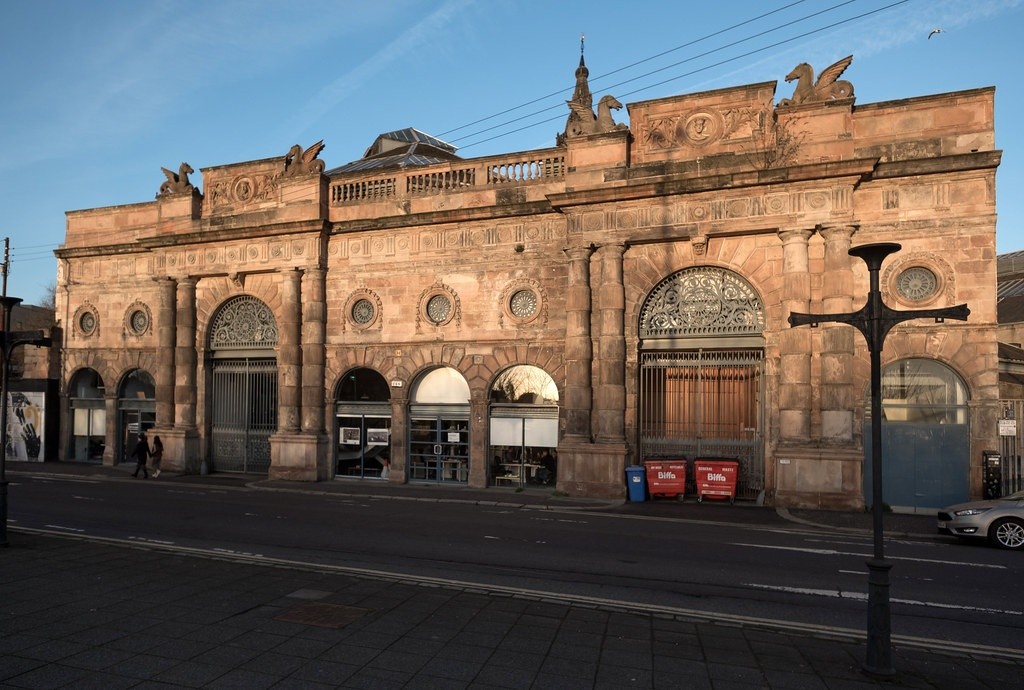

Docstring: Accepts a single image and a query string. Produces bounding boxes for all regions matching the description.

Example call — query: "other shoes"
[143,474,148,479]
[131,473,138,477]
[152,470,160,478]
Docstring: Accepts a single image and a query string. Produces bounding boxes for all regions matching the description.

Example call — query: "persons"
[132,432,151,478]
[507,448,557,481]
[150,435,164,478]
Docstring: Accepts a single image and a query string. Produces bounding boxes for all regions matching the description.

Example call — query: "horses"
[160,162,194,195]
[779,54,854,105]
[564,95,628,135]
[285,139,326,175]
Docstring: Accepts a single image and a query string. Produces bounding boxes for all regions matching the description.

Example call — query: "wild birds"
[928,29,946,40]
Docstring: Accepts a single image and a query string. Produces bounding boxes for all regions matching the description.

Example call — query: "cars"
[937,490,1024,550]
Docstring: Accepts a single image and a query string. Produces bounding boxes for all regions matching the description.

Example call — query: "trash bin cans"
[644,455,688,503]
[693,457,739,504]
[625,465,645,502]
